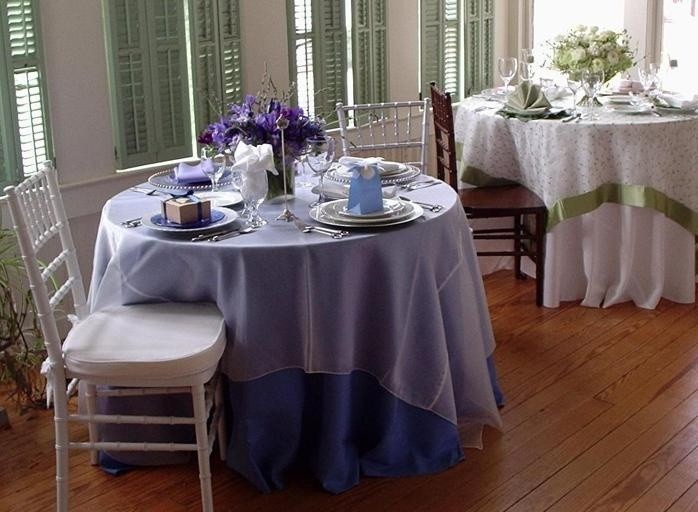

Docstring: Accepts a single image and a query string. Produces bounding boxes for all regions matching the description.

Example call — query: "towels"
[658,88,698,109]
[339,157,400,173]
[497,80,552,122]
[173,159,213,183]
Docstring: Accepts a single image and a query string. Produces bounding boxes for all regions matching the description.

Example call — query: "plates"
[310,199,424,228]
[142,207,238,233]
[193,192,242,207]
[324,161,422,185]
[148,169,233,189]
[655,106,696,114]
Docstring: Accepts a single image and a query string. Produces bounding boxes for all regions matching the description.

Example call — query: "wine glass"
[231,168,269,228]
[305,136,336,208]
[497,57,665,121]
[200,147,226,192]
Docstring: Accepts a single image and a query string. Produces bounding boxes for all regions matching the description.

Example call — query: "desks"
[453,85,698,311]
[83,155,506,493]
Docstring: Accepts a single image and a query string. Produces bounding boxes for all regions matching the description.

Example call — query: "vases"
[265,156,294,204]
[576,84,604,106]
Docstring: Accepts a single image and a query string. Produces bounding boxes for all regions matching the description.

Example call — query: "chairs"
[5,161,227,512]
[336,98,430,174]
[430,82,547,305]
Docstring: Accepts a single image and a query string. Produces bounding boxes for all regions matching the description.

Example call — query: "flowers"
[197,97,324,156]
[546,25,640,84]
